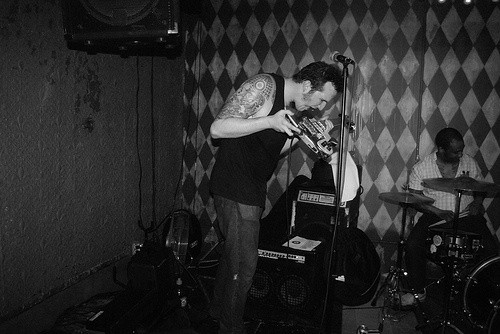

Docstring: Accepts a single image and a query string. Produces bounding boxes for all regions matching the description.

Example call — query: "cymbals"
[379,191,435,203]
[422,176,500,193]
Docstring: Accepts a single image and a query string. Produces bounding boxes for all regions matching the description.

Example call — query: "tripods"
[414,191,466,334]
[371,204,421,334]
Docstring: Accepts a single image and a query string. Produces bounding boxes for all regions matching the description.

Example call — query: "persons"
[207,62,344,334]
[401,127,492,293]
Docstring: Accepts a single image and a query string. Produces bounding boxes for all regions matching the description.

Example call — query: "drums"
[427,229,482,260]
[463,254,500,328]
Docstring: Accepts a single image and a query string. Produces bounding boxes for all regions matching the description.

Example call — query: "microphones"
[338,114,357,132]
[329,50,357,66]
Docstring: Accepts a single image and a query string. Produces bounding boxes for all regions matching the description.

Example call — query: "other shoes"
[400,288,427,306]
[208,314,253,325]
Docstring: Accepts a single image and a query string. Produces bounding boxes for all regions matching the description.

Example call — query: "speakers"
[60,0,183,50]
[245,253,328,331]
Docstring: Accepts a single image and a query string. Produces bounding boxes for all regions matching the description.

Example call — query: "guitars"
[285,107,356,158]
